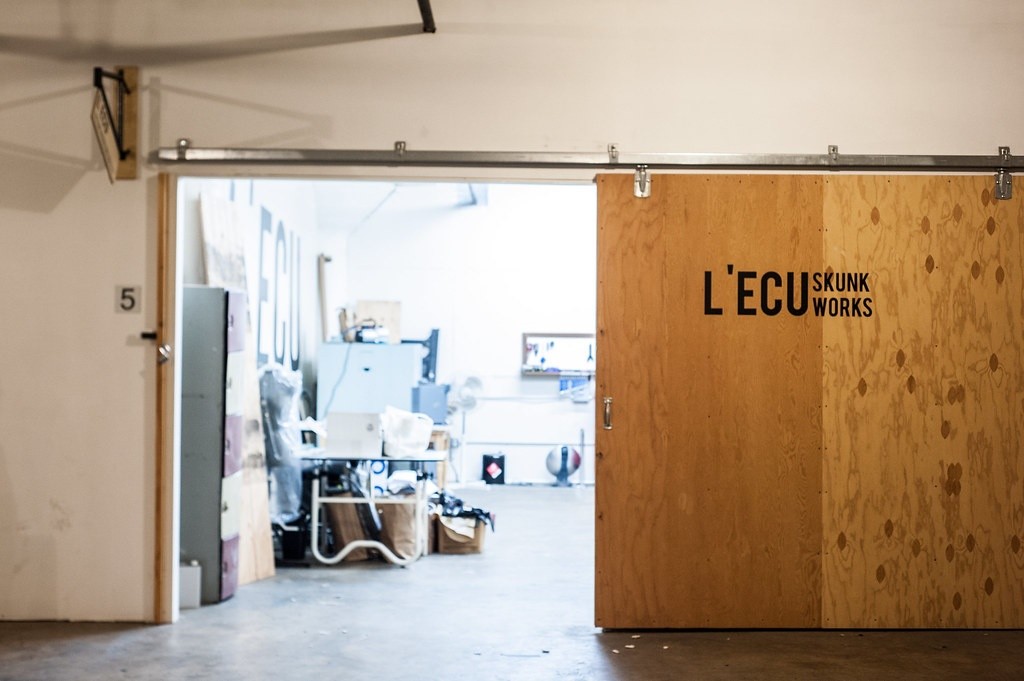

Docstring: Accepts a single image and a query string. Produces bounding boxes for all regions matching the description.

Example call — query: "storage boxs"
[437,514,484,555]
[338,299,402,344]
[324,413,382,457]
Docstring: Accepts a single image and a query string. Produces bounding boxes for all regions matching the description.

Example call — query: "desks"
[294,448,447,565]
